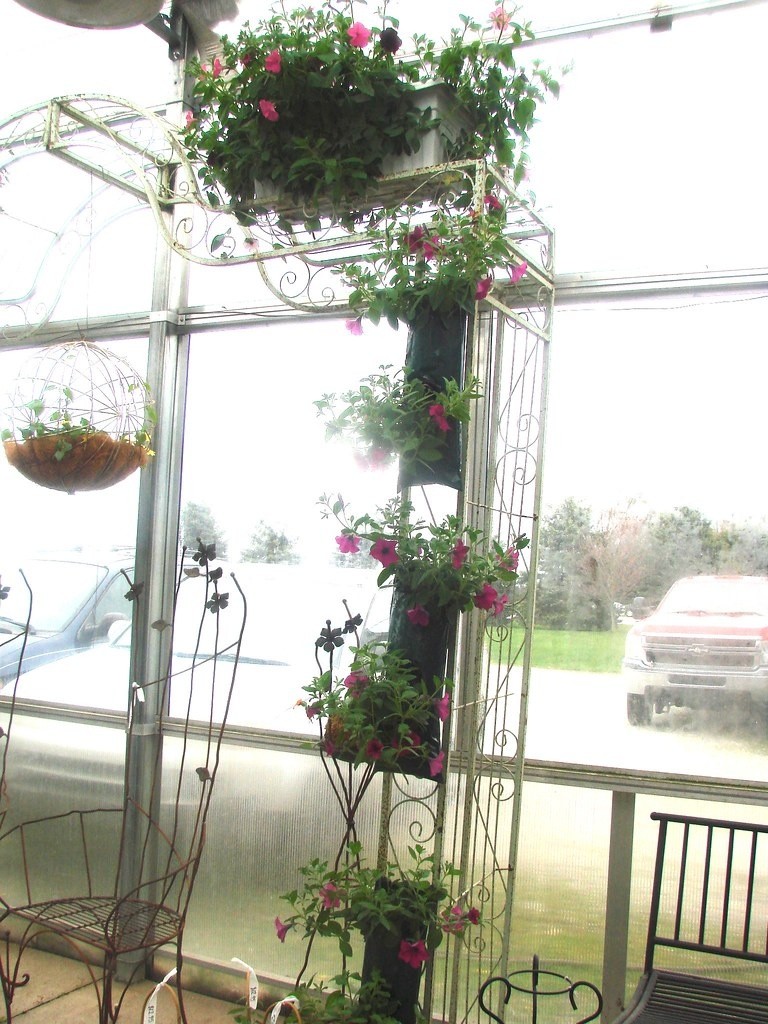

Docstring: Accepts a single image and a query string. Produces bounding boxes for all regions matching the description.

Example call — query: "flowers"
[313,364,485,480]
[180,0,560,335]
[276,841,484,969]
[316,491,531,626]
[300,639,452,780]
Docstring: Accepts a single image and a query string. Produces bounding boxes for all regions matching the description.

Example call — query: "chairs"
[0,537,246,1024]
[612,812,768,1024]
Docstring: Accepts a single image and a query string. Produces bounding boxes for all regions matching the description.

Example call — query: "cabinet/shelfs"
[47,0,612,1024]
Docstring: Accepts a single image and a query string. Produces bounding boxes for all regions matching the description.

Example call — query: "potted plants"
[0,383,157,495]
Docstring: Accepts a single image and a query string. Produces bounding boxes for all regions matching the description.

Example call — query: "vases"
[255,82,481,220]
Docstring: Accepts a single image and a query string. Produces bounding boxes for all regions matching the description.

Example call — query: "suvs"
[624,574,768,726]
[0,537,209,701]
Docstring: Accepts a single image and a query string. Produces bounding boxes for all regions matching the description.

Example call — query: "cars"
[0,567,465,882]
